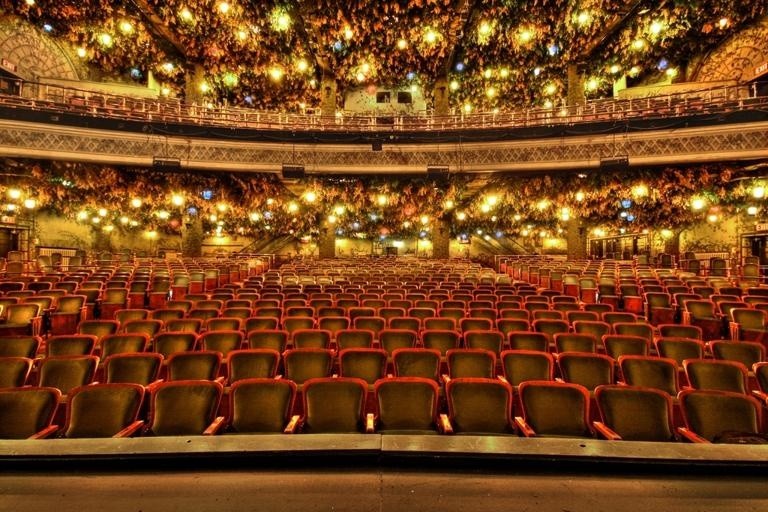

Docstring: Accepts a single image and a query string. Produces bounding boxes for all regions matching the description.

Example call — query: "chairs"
[0,253,768,444]
